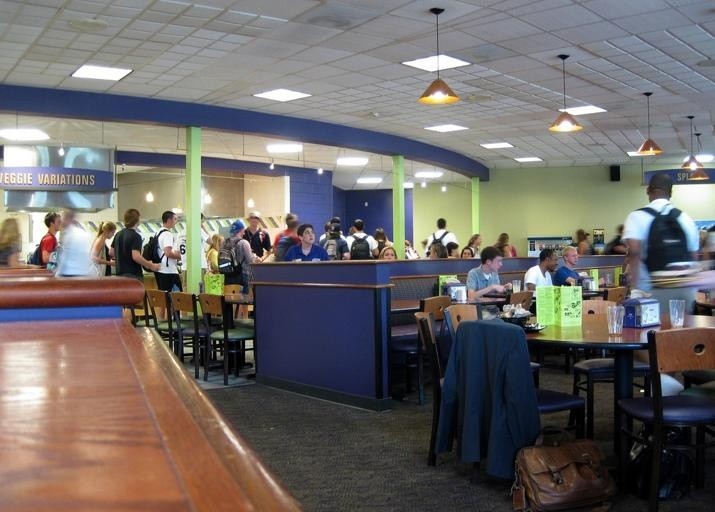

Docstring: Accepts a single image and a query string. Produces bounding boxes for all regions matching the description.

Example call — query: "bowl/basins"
[496,312,534,328]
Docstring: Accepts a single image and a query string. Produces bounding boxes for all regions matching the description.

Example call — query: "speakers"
[609,164,621,182]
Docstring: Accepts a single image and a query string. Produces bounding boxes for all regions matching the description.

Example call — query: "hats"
[229,219,244,234]
[246,211,261,221]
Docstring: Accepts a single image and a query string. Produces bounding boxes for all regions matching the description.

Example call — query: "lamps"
[637,89,664,155]
[145,189,155,203]
[681,115,703,170]
[415,8,463,104]
[688,133,709,181]
[58,143,66,155]
[548,52,583,134]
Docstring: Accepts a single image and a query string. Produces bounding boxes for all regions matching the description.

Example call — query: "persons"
[621,172,698,313]
[200,212,397,321]
[0,219,22,267]
[404,218,519,261]
[576,225,715,271]
[41,209,183,321]
[524,249,558,316]
[465,246,513,300]
[554,245,580,286]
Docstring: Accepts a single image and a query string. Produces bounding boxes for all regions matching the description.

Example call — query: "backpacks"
[217,237,245,274]
[27,246,41,264]
[143,229,172,274]
[351,234,371,259]
[324,236,341,261]
[427,232,448,256]
[637,208,690,272]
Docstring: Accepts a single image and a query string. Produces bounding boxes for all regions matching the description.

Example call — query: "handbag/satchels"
[613,423,695,500]
[514,426,616,512]
[88,253,106,279]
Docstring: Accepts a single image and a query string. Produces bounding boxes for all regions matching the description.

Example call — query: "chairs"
[131,279,255,386]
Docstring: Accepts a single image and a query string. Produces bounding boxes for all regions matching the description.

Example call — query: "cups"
[605,272,615,287]
[608,335,624,343]
[619,273,626,286]
[668,299,686,328]
[454,289,468,301]
[587,281,598,291]
[512,280,521,293]
[607,306,626,335]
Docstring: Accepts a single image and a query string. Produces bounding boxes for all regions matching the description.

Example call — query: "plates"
[524,323,548,333]
